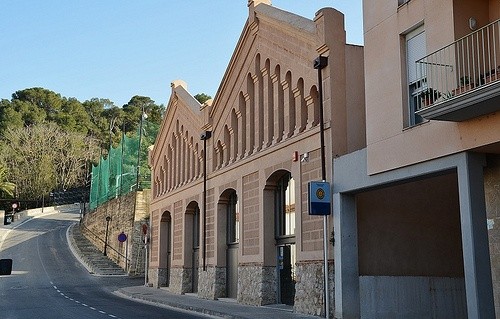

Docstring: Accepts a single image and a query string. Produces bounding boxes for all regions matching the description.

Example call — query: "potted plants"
[483,65,500,84]
[420,87,441,106]
[452,76,477,94]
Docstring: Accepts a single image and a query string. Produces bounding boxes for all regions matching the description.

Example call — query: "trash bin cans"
[12,213,15,222]
[4,212,12,225]
